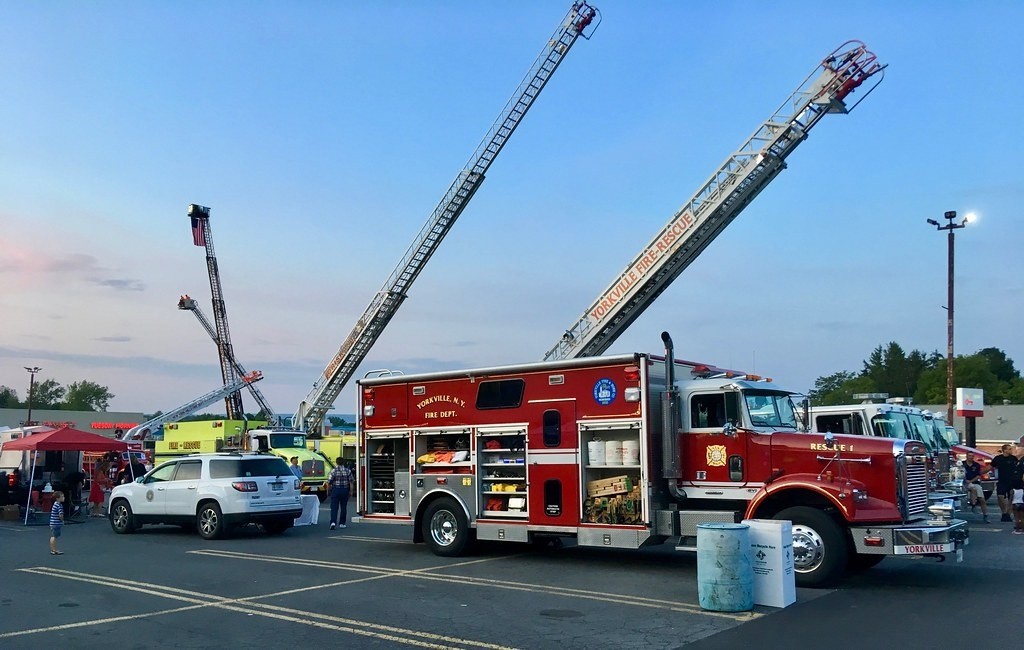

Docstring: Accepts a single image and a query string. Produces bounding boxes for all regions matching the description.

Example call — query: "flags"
[190,217,206,246]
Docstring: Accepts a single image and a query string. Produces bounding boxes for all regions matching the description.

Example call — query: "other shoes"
[1014,524,1019,528]
[1013,527,1024,535]
[1006,513,1012,522]
[983,515,993,524]
[1000,513,1007,522]
[972,499,977,505]
[91,513,107,517]
[50,549,64,555]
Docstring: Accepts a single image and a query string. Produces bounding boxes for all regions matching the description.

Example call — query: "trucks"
[1,425,84,503]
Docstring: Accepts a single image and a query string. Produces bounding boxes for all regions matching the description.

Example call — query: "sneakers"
[339,523,348,529]
[329,522,336,529]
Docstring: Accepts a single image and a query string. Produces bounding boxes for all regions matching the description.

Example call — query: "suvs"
[106,452,303,539]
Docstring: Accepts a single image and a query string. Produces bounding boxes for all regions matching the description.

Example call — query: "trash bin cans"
[696,522,755,612]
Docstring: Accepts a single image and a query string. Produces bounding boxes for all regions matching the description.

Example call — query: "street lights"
[927,209,978,428]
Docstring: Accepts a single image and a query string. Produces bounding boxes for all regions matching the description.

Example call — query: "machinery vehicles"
[121,1,888,501]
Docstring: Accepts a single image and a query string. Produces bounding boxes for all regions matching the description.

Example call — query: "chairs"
[69,497,89,515]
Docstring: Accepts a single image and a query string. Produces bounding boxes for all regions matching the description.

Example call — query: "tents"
[0,426,135,526]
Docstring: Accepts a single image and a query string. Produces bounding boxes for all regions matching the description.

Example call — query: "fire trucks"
[354,330,998,591]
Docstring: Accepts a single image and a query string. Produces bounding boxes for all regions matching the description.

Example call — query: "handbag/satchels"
[99,484,111,494]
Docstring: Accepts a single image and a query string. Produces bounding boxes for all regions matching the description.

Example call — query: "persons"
[144,459,152,473]
[90,462,113,517]
[980,445,1018,522]
[1012,444,1024,535]
[95,451,123,471]
[289,457,303,482]
[50,491,65,555]
[962,451,991,524]
[124,455,147,484]
[115,427,123,438]
[121,473,129,484]
[327,457,355,529]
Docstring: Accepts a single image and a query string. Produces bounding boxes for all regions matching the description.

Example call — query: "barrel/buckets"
[588,440,606,466]
[606,441,623,465]
[696,523,754,611]
[622,440,639,465]
